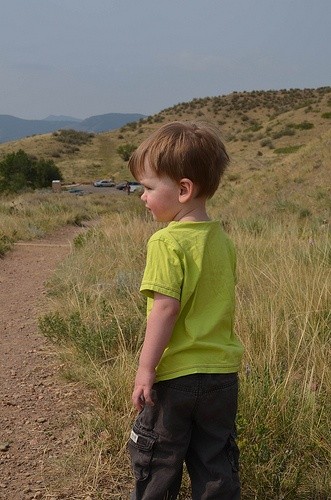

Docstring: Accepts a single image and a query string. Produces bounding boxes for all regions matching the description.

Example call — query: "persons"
[125,122,244,500]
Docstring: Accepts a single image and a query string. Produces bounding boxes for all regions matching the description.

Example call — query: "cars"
[70,187,84,196]
[95,179,143,192]
